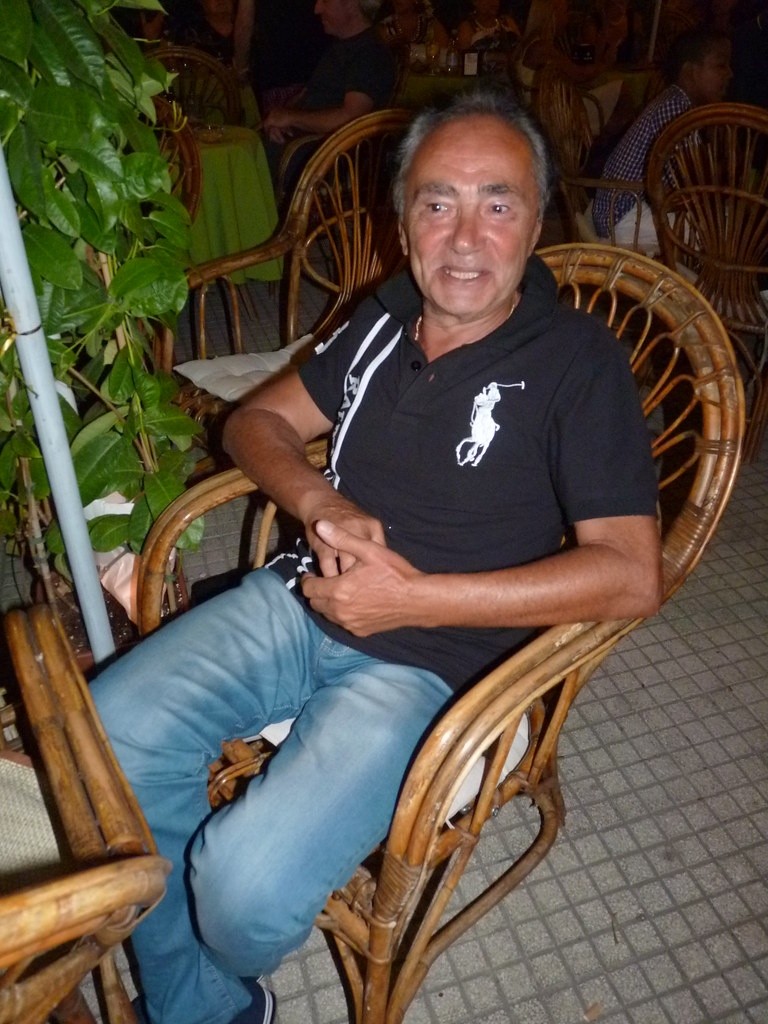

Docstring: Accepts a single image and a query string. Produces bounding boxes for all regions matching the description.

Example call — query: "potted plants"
[0,0,212,671]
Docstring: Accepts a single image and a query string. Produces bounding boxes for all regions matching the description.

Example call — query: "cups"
[198,112,223,143]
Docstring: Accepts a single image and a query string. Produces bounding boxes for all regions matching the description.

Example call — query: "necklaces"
[670,84,692,106]
[474,17,500,32]
[395,9,421,42]
[601,7,625,26]
[415,304,515,340]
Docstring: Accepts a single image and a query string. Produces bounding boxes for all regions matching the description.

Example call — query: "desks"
[184,77,259,128]
[592,62,668,113]
[191,122,284,282]
[396,68,515,110]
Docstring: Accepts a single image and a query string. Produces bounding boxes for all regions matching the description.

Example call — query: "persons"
[87,88,666,1024]
[111,0,768,340]
[579,25,734,383]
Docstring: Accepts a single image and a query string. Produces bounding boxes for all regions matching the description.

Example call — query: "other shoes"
[229,979,275,1024]
[130,973,264,1024]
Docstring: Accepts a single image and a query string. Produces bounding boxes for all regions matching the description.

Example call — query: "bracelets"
[756,13,765,32]
[484,51,488,65]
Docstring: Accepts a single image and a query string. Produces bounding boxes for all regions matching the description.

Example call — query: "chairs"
[138,243,748,1024]
[0,601,171,1024]
[105,0,768,479]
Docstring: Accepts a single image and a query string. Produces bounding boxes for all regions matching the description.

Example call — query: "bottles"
[425,17,441,76]
[446,30,460,77]
